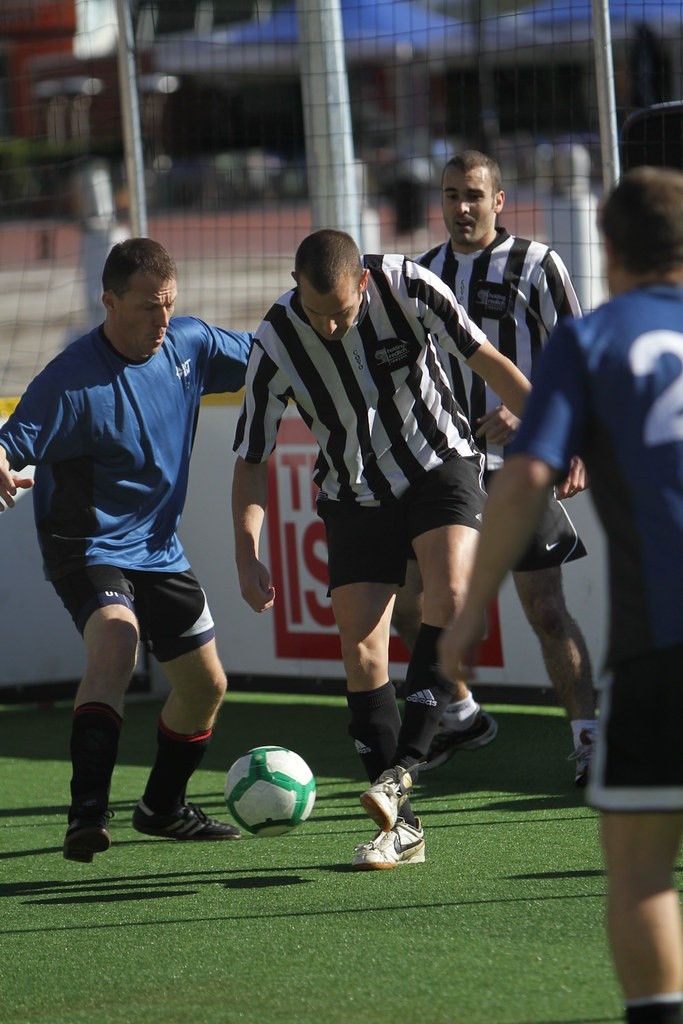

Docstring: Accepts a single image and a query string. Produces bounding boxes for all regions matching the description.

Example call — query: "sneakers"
[131,794,242,840]
[567,743,595,788]
[63,806,114,863]
[351,816,426,870]
[417,702,499,772]
[359,761,428,833]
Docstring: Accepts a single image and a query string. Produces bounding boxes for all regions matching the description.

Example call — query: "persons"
[409,171,683,1024]
[0,237,255,862]
[232,227,586,868]
[391,153,594,782]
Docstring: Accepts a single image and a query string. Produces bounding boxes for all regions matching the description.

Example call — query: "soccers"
[223,745,317,838]
